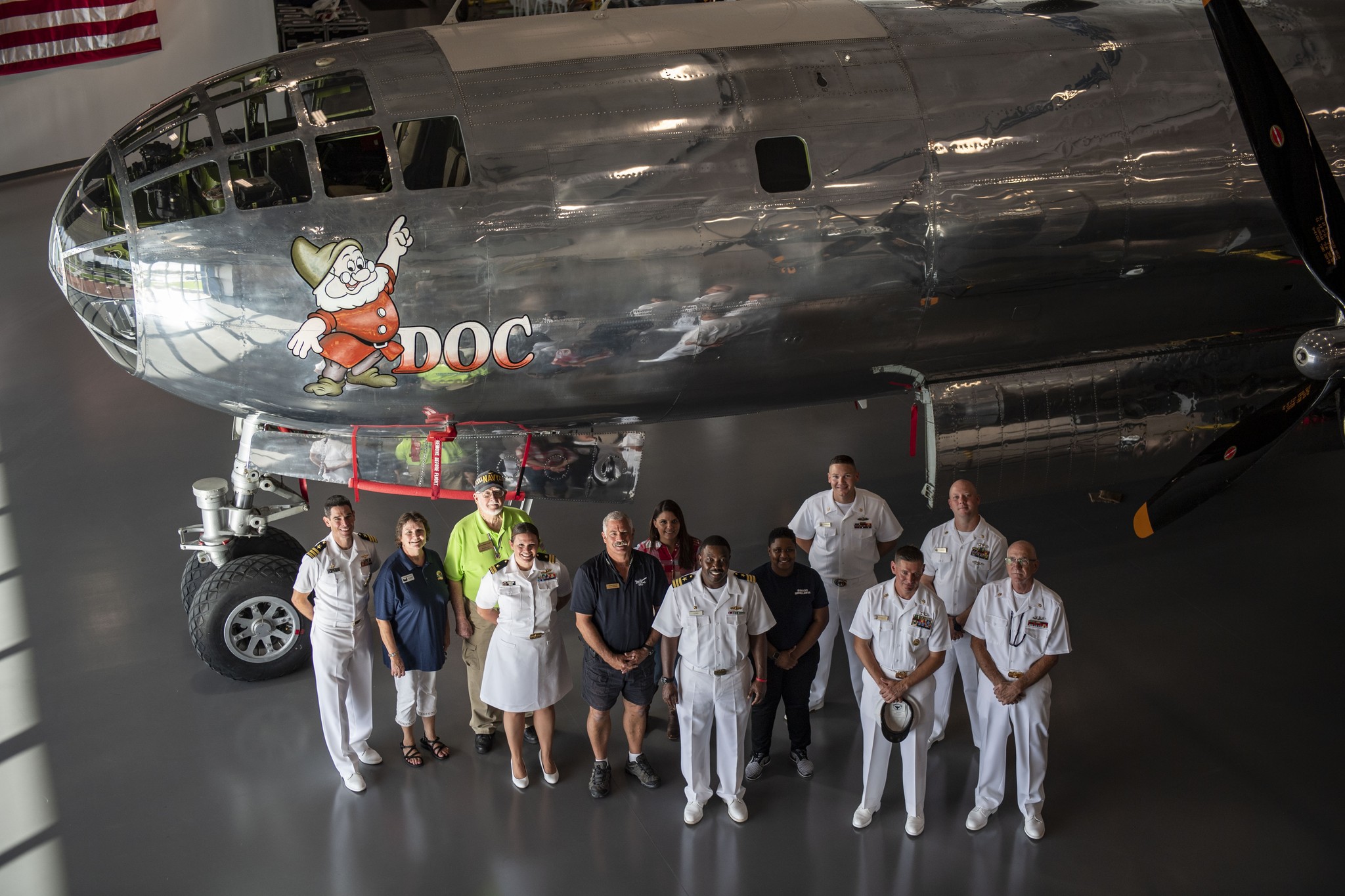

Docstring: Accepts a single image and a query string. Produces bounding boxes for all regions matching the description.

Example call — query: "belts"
[822,575,867,587]
[879,663,915,680]
[503,628,549,640]
[680,656,746,676]
[318,617,364,628]
[996,665,1025,679]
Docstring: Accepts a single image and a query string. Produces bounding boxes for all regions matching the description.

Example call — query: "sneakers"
[745,750,771,780]
[788,748,814,777]
[625,753,662,788]
[588,761,612,798]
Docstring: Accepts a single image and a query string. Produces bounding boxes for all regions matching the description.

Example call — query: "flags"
[0,0,162,76]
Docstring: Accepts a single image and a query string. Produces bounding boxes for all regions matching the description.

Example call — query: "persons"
[372,511,451,768]
[745,526,829,780]
[630,499,701,739]
[849,545,953,837]
[395,435,469,490]
[651,535,778,825]
[570,511,670,798]
[536,282,781,375]
[308,428,359,484]
[444,469,548,755]
[291,495,382,793]
[476,522,572,788]
[918,479,1008,749]
[784,454,904,720]
[514,435,597,503]
[966,540,1072,841]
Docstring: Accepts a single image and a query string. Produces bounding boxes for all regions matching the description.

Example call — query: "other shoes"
[666,709,680,739]
[785,699,824,720]
[523,725,539,744]
[474,732,494,754]
[927,732,945,750]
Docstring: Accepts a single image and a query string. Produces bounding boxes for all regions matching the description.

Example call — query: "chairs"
[441,146,467,187]
[327,120,423,197]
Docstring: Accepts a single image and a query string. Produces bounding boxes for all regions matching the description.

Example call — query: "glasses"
[476,490,505,498]
[1005,557,1034,567]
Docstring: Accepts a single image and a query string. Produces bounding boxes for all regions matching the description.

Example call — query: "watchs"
[661,676,675,684]
[953,616,961,633]
[643,644,656,656]
[770,650,781,663]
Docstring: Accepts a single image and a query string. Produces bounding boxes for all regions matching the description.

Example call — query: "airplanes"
[46,0,1344,681]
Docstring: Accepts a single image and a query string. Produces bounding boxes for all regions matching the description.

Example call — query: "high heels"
[510,757,529,789]
[538,749,559,784]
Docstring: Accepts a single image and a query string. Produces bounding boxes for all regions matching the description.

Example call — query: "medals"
[494,553,501,559]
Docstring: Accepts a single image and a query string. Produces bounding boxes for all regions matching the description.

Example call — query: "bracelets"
[756,677,768,682]
[388,650,400,657]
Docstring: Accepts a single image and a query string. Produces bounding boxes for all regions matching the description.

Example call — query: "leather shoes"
[684,799,708,824]
[966,805,998,830]
[1025,814,1044,839]
[905,811,925,835]
[339,771,366,791]
[357,746,382,765]
[852,802,881,828]
[721,796,748,823]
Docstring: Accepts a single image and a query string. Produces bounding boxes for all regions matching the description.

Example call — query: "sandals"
[420,732,449,760]
[400,739,423,767]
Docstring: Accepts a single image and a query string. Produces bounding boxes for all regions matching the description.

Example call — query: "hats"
[474,469,505,492]
[874,692,921,743]
